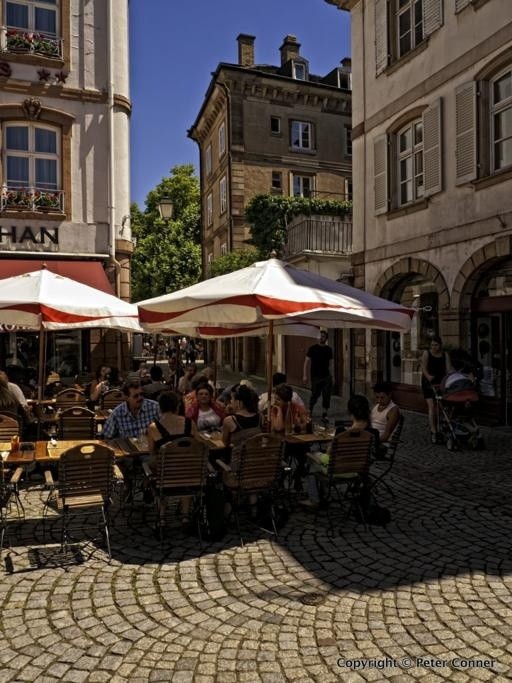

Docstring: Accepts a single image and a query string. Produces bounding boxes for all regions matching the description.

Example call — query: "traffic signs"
[157,197,174,226]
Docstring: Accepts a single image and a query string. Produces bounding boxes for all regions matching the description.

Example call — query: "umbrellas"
[134,249,414,432]
[0,262,156,398]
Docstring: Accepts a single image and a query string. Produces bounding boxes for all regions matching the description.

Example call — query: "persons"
[302,330,335,424]
[258,372,303,411]
[90,366,109,403]
[138,335,205,364]
[105,380,160,485]
[149,393,206,530]
[215,384,265,522]
[419,334,453,444]
[137,363,146,379]
[178,364,198,392]
[268,384,307,431]
[186,382,226,431]
[145,365,171,398]
[104,366,128,395]
[368,381,400,443]
[299,394,386,507]
[199,366,221,397]
[162,357,182,384]
[0,372,30,420]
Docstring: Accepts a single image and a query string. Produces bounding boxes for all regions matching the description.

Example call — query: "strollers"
[430,372,487,451]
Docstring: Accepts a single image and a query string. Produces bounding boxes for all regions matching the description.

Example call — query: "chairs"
[141,437,210,554]
[367,413,405,499]
[100,388,124,410]
[0,454,27,558]
[43,442,124,565]
[58,406,97,441]
[305,427,375,539]
[54,388,87,413]
[0,410,23,442]
[216,433,286,548]
